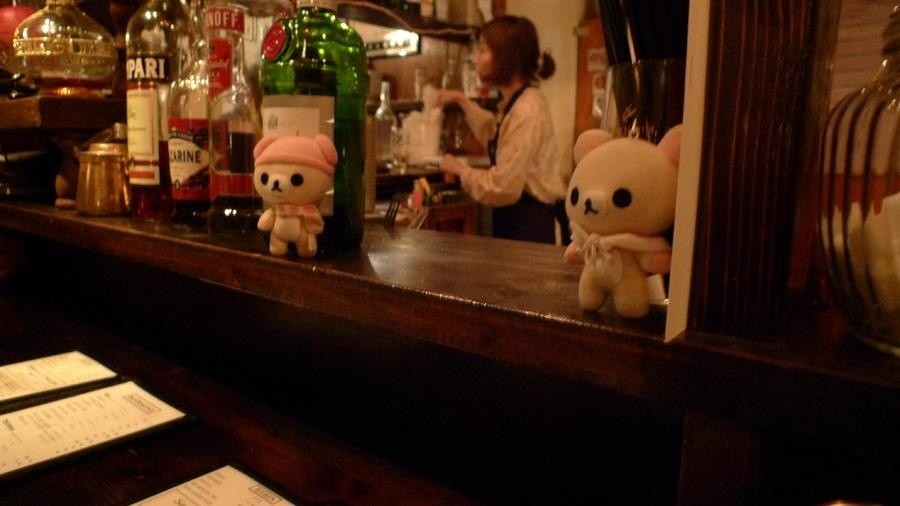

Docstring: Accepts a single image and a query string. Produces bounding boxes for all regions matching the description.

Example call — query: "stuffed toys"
[564,120,684,328]
[250,133,341,257]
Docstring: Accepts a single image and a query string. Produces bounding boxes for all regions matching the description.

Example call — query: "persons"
[420,17,571,247]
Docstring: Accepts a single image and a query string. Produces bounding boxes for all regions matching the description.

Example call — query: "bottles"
[204,1,270,249]
[166,1,214,223]
[257,1,366,260]
[123,1,201,223]
[364,56,500,215]
[74,142,138,218]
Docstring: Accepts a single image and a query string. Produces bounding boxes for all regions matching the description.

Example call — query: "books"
[0,345,193,485]
[121,459,312,506]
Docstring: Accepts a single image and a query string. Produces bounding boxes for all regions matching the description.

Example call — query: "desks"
[0,293,481,506]
